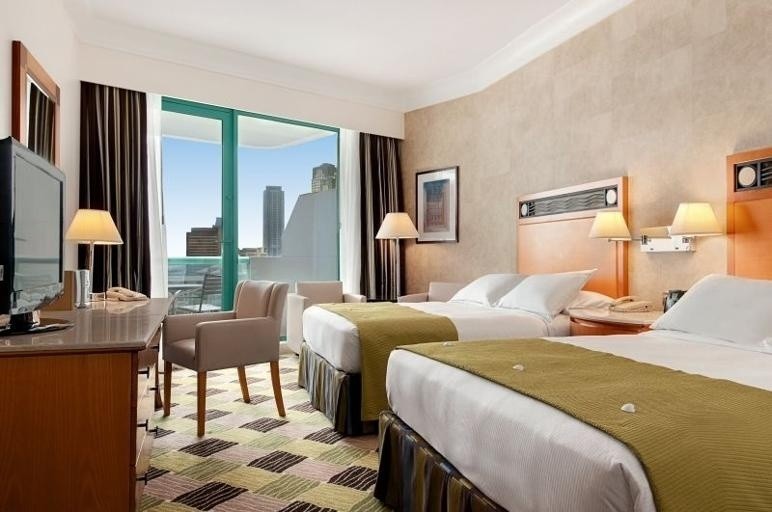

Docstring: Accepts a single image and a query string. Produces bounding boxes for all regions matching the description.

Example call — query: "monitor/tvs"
[0,136,75,338]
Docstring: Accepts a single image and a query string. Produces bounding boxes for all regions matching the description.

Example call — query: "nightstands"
[568,310,653,336]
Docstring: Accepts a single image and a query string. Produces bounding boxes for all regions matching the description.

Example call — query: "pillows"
[494,268,601,322]
[448,272,528,306]
[650,271,772,350]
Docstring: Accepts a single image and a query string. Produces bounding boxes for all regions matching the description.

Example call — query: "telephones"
[106,287,147,302]
[609,296,654,313]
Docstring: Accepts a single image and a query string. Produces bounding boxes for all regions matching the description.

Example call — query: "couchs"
[393,281,463,305]
[287,281,367,358]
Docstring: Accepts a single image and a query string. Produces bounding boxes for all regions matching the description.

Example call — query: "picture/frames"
[416,164,459,243]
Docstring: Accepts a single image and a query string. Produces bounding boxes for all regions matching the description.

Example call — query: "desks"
[0,293,180,509]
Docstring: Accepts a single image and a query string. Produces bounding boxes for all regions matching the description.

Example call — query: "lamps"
[592,200,723,259]
[376,208,420,302]
[65,208,123,293]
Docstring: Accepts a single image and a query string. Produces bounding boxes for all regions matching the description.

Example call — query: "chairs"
[164,280,289,440]
[169,265,222,312]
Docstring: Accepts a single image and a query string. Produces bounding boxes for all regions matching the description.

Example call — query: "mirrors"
[10,37,63,171]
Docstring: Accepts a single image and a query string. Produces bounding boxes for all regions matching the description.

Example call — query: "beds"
[380,145,772,512]
[296,174,629,430]
